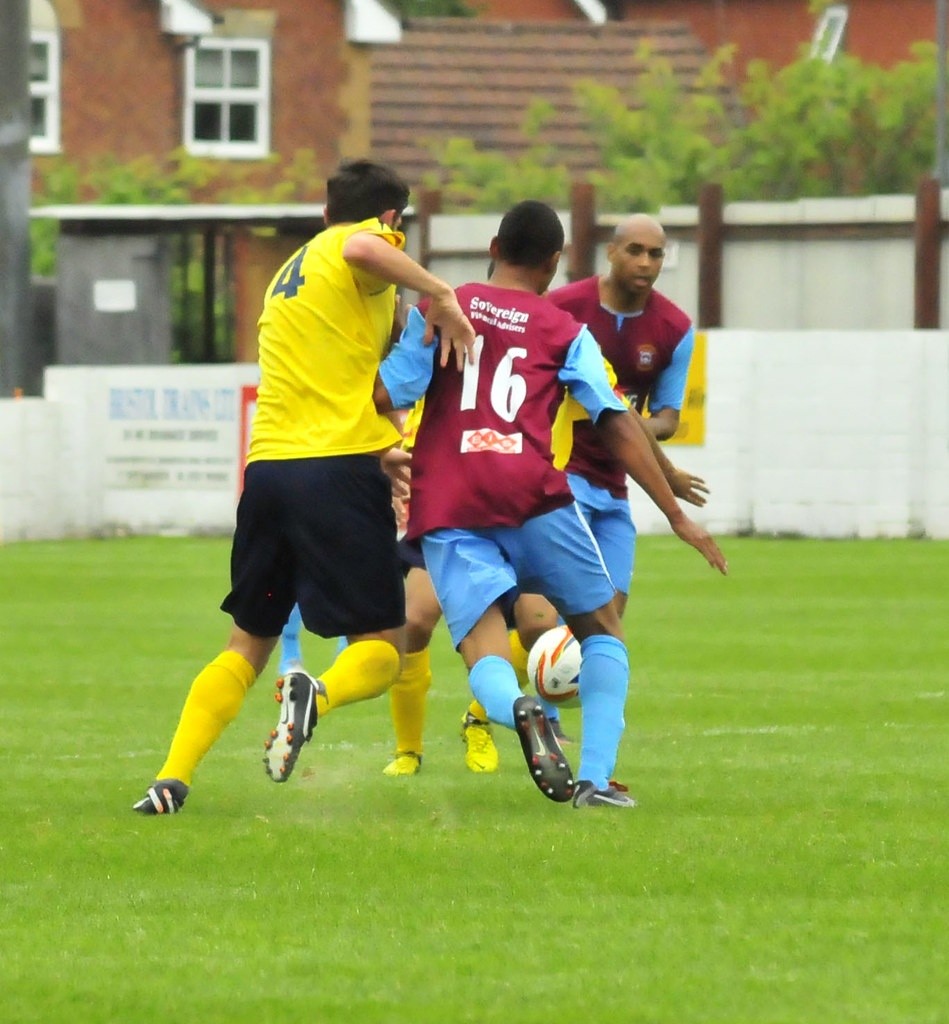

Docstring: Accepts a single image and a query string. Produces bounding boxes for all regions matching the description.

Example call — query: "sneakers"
[571,781,637,809]
[549,716,570,746]
[263,672,320,783]
[462,711,499,773]
[383,749,422,775]
[133,784,185,815]
[514,695,574,802]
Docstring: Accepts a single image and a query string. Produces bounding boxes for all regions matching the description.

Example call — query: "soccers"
[526,624,583,703]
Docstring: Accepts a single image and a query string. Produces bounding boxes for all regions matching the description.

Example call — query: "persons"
[382,260,710,776]
[278,601,349,676]
[132,162,476,814]
[374,200,728,809]
[545,212,695,745]
